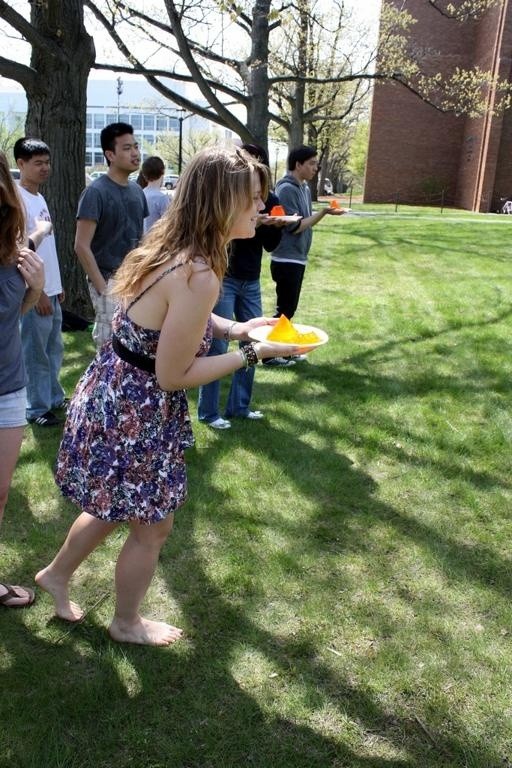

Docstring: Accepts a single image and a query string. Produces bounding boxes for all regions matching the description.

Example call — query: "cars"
[8,168,21,186]
[86,171,179,191]
[303,178,334,196]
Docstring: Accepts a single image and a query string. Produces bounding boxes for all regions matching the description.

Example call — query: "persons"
[13,138,73,426]
[136,155,171,240]
[32,143,318,649]
[197,141,299,429]
[137,169,148,188]
[262,144,348,369]
[1,148,45,610]
[73,121,151,356]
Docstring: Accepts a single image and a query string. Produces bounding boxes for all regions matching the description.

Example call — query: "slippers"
[0,583,36,607]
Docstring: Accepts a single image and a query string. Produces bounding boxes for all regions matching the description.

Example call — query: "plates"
[320,205,352,215]
[247,323,329,360]
[264,215,302,226]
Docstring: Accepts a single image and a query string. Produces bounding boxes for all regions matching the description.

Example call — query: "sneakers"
[248,410,264,419]
[210,418,231,429]
[289,354,306,361]
[264,357,296,368]
[57,397,71,409]
[31,411,61,426]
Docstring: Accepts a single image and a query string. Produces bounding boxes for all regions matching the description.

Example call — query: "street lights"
[176,106,186,175]
[274,147,279,186]
[116,76,123,124]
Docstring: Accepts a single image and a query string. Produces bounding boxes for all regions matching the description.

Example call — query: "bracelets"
[222,318,239,344]
[236,341,262,370]
